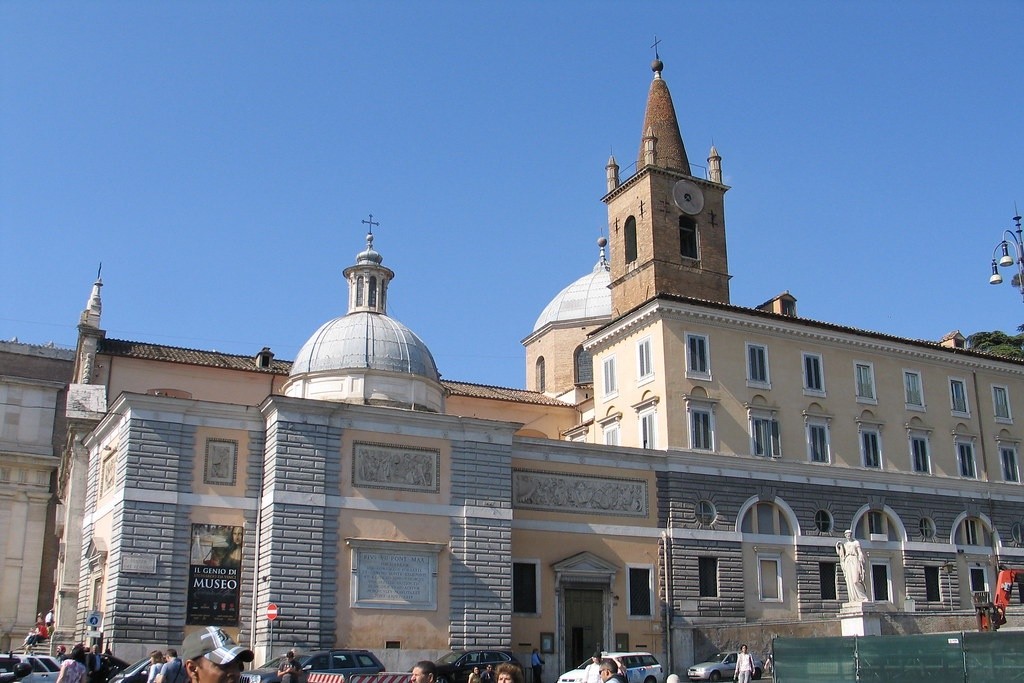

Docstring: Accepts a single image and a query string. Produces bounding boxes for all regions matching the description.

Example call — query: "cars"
[0,652,182,683]
[686,651,764,683]
[406,650,524,683]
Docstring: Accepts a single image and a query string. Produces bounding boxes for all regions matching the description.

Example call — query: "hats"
[181,625,254,665]
[592,652,602,659]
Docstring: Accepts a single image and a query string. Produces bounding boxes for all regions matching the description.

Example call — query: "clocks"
[673,179,705,214]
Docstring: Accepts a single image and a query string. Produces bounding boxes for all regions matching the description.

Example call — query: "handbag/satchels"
[749,654,753,672]
[536,654,545,665]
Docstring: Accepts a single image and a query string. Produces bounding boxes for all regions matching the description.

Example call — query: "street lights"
[942,560,953,611]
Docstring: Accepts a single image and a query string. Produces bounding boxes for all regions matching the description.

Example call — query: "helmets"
[57,645,66,654]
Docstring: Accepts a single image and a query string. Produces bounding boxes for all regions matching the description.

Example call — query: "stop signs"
[266,604,278,620]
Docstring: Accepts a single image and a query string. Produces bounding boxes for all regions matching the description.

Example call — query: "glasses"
[599,668,612,675]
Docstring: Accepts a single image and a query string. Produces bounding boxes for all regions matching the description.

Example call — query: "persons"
[278,651,302,683]
[24,645,34,656]
[531,648,545,683]
[985,602,1007,631]
[494,661,525,683]
[147,649,187,683]
[468,665,481,683]
[45,608,55,626]
[25,612,52,646]
[613,656,627,676]
[105,649,112,656]
[599,658,628,683]
[411,661,438,683]
[835,530,868,602]
[56,645,68,664]
[582,651,604,683]
[481,664,495,683]
[733,644,755,683]
[183,626,254,683]
[55,643,86,683]
[86,644,103,683]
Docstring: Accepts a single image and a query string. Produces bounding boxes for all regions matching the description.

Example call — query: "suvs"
[238,649,385,683]
[557,652,664,683]
